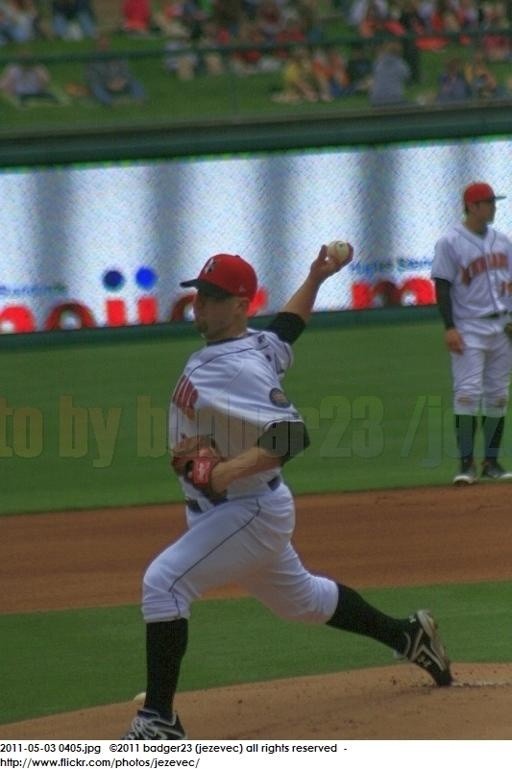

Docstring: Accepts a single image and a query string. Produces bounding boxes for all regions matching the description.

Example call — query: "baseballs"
[325,240,349,263]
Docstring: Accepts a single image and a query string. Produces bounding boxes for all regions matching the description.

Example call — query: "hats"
[180,255,257,297]
[465,184,507,208]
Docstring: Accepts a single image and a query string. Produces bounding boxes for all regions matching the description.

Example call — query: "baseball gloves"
[170,435,227,506]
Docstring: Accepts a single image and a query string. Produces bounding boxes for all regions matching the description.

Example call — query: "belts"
[184,474,281,512]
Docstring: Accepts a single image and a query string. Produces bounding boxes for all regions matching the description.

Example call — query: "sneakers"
[120,709,187,740]
[453,467,476,483]
[401,610,451,687]
[481,461,512,482]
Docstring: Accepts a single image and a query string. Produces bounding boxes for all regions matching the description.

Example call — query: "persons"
[1,1,511,110]
[429,182,511,485]
[115,238,453,738]
